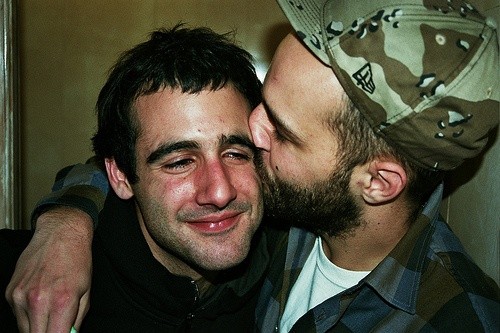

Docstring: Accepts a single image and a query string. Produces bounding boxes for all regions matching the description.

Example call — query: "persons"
[5,0,500,333]
[0,20,272,333]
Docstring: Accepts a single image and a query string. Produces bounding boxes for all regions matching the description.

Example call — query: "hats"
[277,0,500,173]
